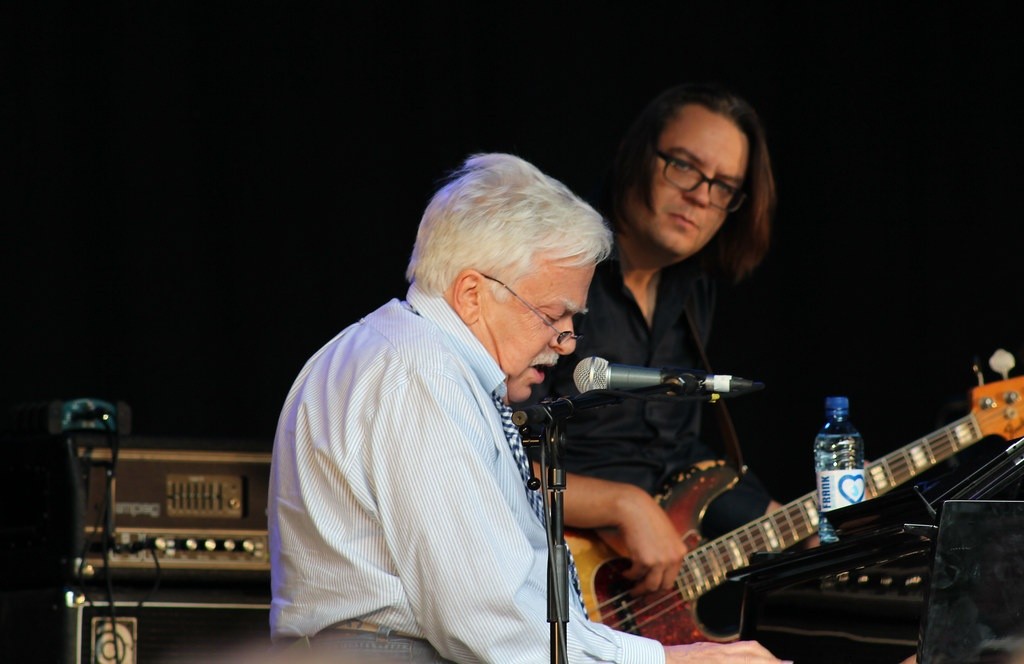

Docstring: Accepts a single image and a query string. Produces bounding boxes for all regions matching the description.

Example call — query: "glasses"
[654,147,748,214]
[483,273,586,354]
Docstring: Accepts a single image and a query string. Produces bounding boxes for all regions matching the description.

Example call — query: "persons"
[509,87,820,590]
[267,153,790,664]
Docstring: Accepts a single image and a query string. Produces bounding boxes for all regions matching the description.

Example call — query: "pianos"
[737,432,1023,664]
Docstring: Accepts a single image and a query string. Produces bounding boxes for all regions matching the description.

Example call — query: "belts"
[326,620,414,642]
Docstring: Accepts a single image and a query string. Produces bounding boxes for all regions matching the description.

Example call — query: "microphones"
[571,356,761,394]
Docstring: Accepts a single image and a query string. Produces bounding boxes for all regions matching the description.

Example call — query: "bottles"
[815,397,864,544]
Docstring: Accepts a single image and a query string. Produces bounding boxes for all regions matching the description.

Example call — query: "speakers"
[59,589,277,664]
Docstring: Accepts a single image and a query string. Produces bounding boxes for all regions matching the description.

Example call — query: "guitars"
[559,347,1024,648]
